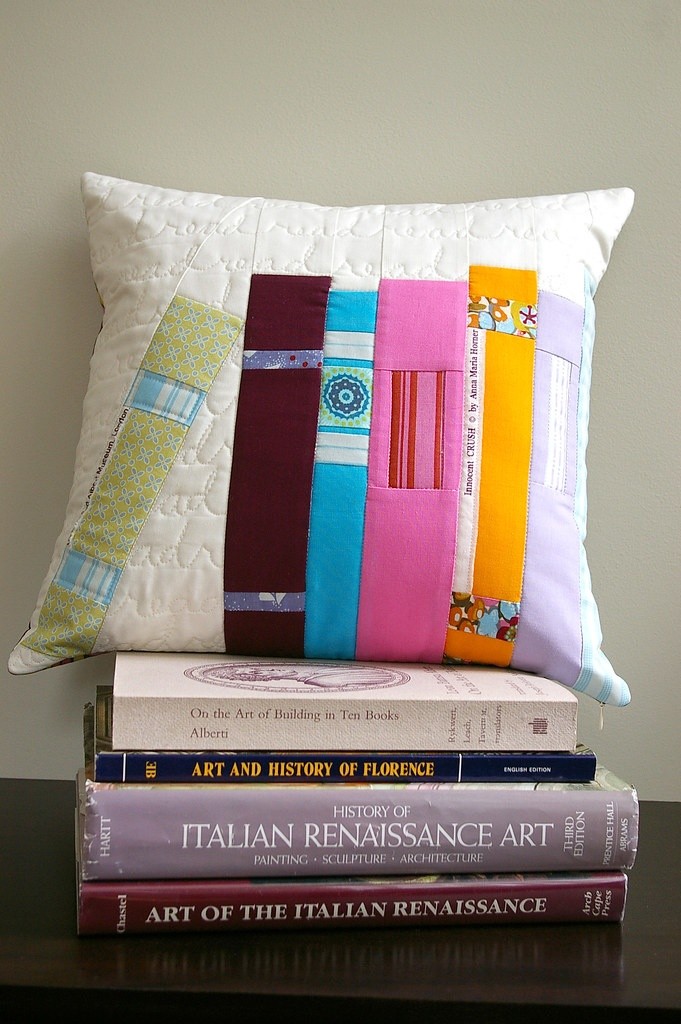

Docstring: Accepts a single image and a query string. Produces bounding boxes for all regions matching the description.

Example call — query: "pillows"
[6,171,638,713]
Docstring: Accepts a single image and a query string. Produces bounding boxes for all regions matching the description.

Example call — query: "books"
[72,652,645,935]
